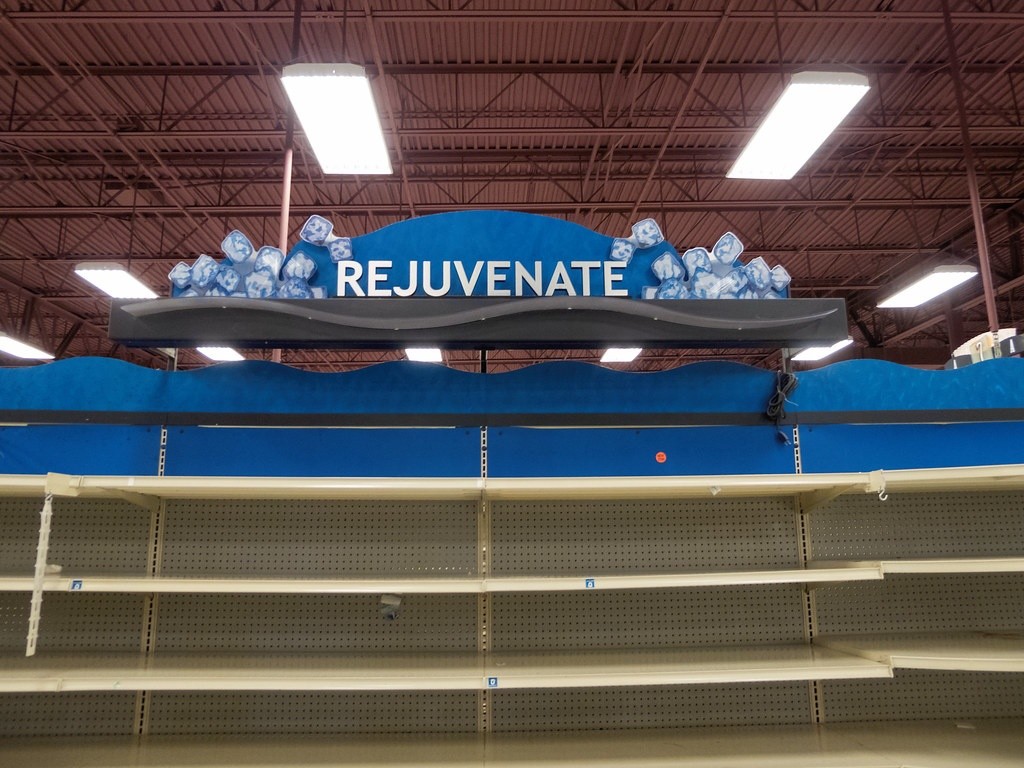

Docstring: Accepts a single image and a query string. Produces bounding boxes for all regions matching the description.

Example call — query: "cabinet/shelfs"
[0,461,1024,768]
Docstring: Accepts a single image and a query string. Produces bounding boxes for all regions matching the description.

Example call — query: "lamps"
[790,331,860,361]
[597,346,644,365]
[193,345,248,360]
[71,159,165,299]
[0,259,59,361]
[873,164,981,309]
[281,0,397,180]
[722,18,877,182]
[403,346,447,362]
